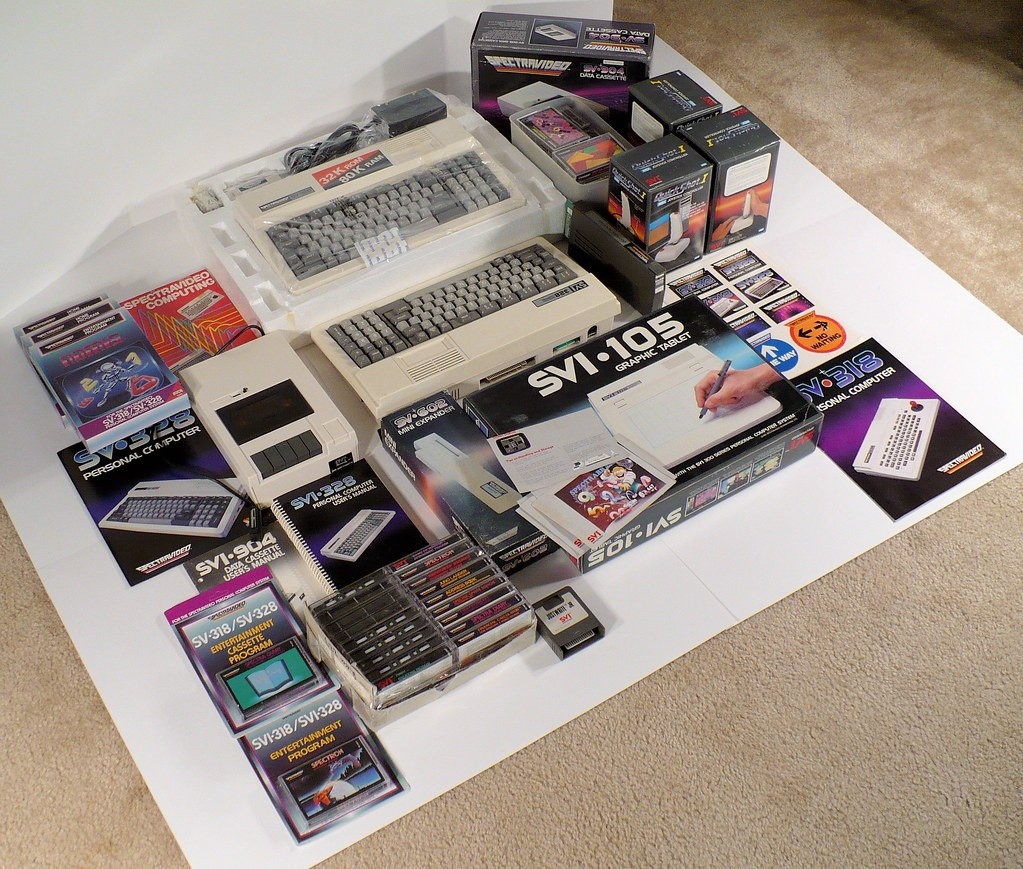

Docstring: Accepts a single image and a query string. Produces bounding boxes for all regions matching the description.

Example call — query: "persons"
[695,362,784,412]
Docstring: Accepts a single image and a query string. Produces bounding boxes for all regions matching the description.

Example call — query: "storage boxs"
[469,10,780,274]
[309,529,538,732]
[376,390,560,576]
[463,294,824,575]
[12,291,190,455]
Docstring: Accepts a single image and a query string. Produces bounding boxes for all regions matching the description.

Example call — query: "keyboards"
[98,478,248,537]
[311,237,622,423]
[236,115,526,296]
[320,509,397,562]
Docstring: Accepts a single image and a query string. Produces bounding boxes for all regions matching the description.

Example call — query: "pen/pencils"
[698,359,732,419]
